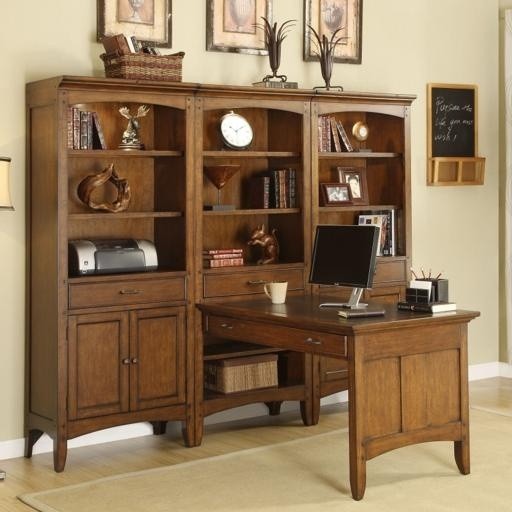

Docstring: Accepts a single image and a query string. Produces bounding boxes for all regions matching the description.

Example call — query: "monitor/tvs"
[309,224,380,307]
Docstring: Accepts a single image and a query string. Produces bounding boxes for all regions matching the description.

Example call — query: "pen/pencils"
[410,267,441,280]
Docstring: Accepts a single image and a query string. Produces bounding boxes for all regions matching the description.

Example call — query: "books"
[67,105,107,150]
[98,34,162,56]
[203,247,244,268]
[241,166,297,211]
[317,113,354,152]
[396,298,458,315]
[357,209,395,257]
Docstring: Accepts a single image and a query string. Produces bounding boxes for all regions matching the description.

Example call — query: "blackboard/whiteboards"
[428,83,479,156]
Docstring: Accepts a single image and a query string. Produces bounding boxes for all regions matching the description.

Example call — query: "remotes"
[337,308,386,319]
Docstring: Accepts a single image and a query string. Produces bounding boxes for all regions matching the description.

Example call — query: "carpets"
[16,403,512,512]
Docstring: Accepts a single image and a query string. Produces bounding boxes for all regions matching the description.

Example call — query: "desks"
[201,295,481,501]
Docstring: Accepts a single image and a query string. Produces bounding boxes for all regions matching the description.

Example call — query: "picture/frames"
[96,0,172,49]
[337,166,369,205]
[303,0,363,65]
[206,0,273,56]
[320,183,354,207]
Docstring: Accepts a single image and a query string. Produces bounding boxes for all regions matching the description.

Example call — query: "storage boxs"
[204,354,279,393]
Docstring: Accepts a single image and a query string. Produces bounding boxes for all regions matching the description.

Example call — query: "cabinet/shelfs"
[310,92,414,428]
[26,75,192,469]
[191,83,311,447]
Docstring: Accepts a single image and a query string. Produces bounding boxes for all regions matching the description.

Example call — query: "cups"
[264,304,287,318]
[263,280,290,303]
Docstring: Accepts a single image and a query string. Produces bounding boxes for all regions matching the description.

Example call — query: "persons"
[329,186,347,202]
[346,173,359,199]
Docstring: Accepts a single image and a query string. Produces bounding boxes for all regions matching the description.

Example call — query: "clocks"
[219,110,253,150]
